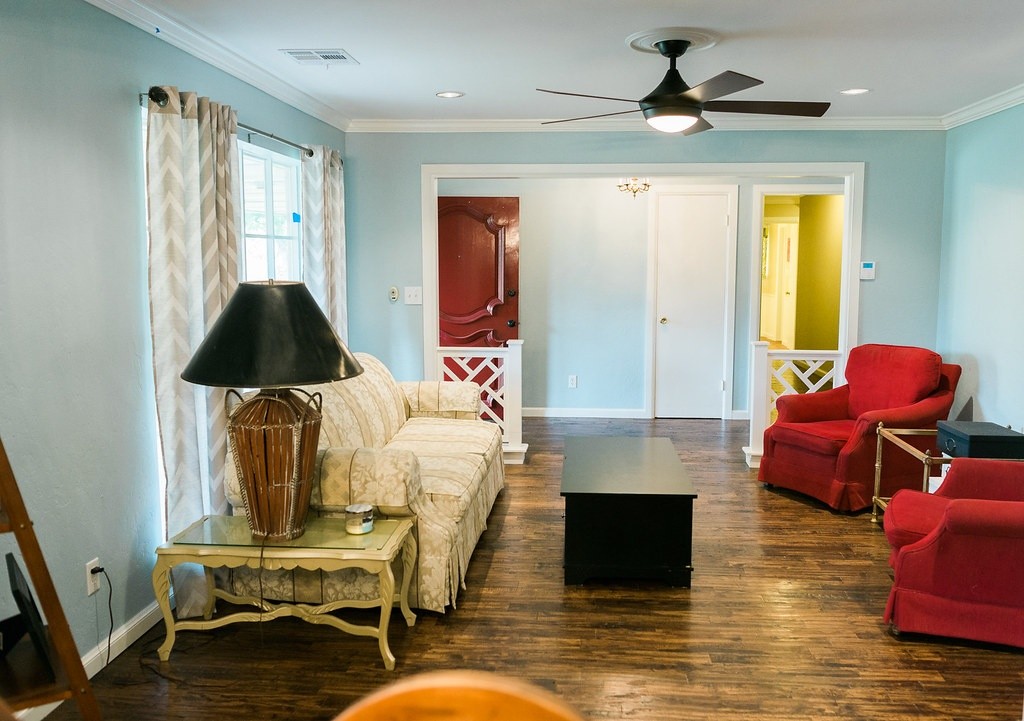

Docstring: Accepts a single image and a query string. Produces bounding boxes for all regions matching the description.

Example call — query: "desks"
[871,422,951,523]
[560,434,698,587]
[151,515,416,670]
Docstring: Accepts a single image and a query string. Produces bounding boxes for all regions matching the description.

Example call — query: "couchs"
[758,345,962,512]
[883,456,1023,650]
[223,352,507,615]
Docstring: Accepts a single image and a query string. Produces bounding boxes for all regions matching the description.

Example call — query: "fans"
[537,40,830,135]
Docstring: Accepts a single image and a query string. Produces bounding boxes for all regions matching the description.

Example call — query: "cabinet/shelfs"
[0,447,101,721]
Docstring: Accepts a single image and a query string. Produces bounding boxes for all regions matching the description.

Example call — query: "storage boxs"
[936,420,1024,459]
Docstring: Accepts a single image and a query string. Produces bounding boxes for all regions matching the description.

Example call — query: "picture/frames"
[0,553,56,682]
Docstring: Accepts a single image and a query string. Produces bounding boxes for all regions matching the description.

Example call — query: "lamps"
[181,279,365,539]
[644,107,700,133]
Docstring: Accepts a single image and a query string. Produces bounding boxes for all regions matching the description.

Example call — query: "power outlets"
[569,375,576,389]
[86,557,100,596]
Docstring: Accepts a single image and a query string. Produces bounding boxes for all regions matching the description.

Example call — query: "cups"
[345,504,374,535]
